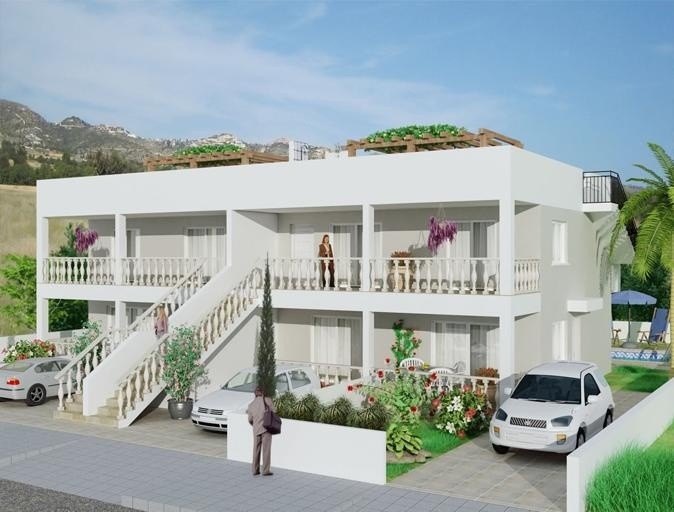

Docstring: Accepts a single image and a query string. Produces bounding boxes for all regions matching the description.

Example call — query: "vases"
[478,384,496,402]
[388,266,415,291]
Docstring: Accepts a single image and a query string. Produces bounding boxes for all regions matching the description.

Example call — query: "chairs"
[400,358,468,391]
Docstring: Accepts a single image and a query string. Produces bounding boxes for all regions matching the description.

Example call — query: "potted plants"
[159,324,209,420]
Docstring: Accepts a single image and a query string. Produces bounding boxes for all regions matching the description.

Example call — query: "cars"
[190,361,322,435]
[0,356,86,407]
[488,358,616,458]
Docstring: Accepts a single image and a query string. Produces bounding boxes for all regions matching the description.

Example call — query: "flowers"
[392,250,413,267]
[1,339,57,364]
[74,227,98,253]
[392,318,422,368]
[426,215,457,255]
[475,367,499,385]
